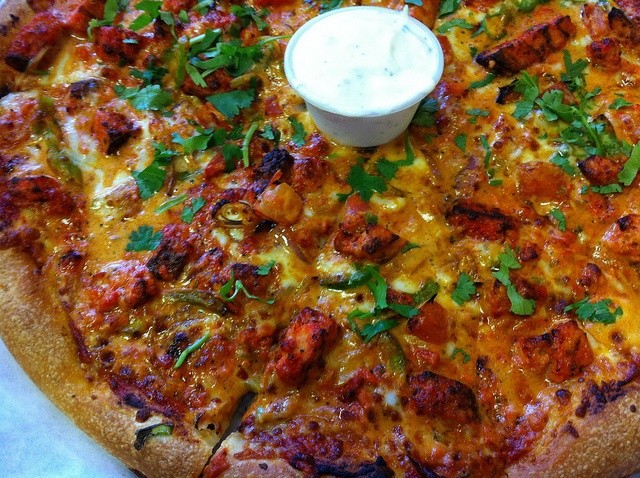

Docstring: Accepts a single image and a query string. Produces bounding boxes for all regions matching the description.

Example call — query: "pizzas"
[0,0,639,477]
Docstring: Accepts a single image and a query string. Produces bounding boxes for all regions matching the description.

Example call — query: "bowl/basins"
[284,4,445,147]
